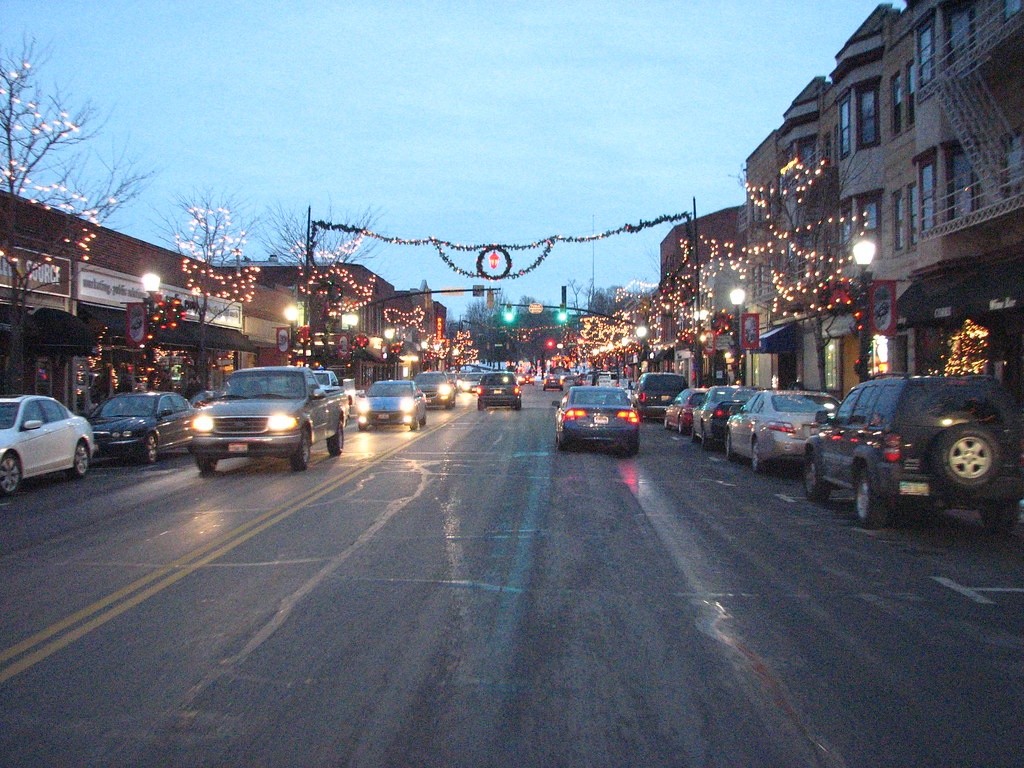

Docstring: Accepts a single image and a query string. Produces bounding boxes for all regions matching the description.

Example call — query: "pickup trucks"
[185,366,347,474]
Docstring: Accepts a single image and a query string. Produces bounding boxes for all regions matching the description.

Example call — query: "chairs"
[245,381,263,397]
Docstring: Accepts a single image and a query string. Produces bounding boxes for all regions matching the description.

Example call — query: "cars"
[724,389,841,473]
[86,389,197,465]
[690,385,761,452]
[476,370,522,412]
[0,393,95,496]
[551,386,640,455]
[311,366,338,390]
[446,363,621,394]
[663,388,709,436]
[357,379,425,431]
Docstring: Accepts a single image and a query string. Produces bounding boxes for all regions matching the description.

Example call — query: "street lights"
[728,287,747,386]
[851,238,876,384]
[140,272,160,391]
[283,301,461,375]
[588,309,715,388]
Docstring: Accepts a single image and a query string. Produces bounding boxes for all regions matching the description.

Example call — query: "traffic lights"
[505,305,514,322]
[556,303,567,323]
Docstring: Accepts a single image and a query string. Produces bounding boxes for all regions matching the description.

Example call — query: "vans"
[627,371,689,423]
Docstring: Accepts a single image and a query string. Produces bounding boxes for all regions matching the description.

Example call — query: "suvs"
[800,369,1023,534]
[413,371,455,410]
